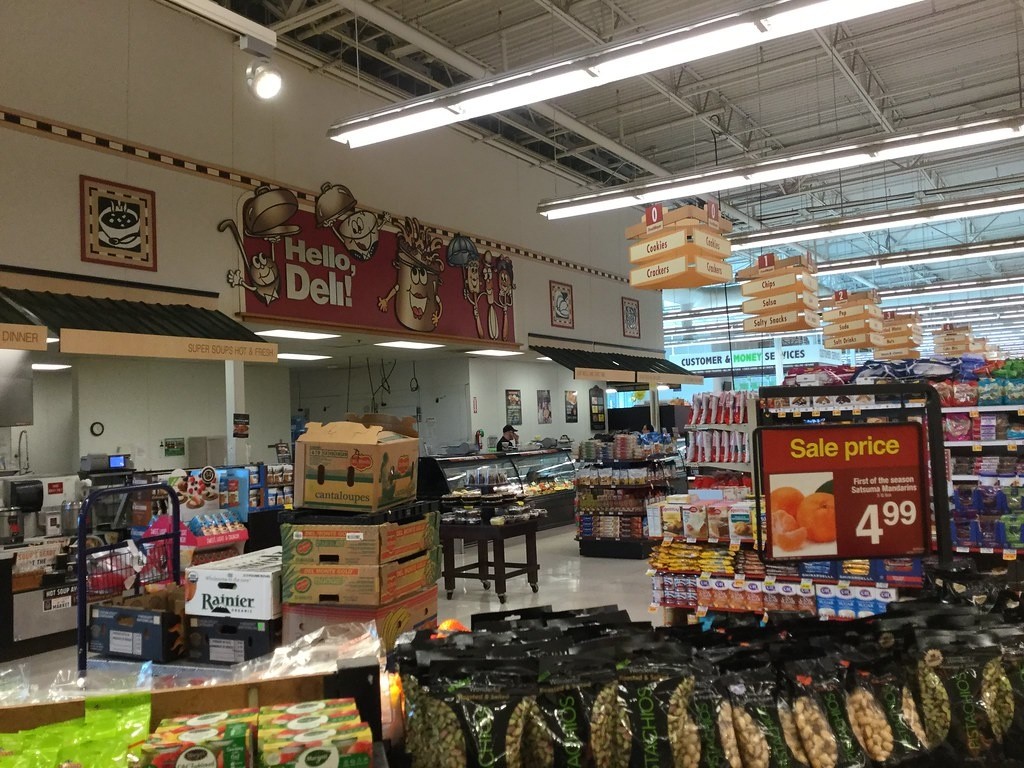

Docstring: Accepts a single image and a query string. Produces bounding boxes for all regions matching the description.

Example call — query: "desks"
[440,517,540,604]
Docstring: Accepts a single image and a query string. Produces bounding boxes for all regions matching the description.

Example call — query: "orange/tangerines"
[770,486,836,552]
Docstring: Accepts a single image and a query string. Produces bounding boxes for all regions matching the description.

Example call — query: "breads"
[664,519,751,537]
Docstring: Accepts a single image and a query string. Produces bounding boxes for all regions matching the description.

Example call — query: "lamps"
[158,0,1024,365]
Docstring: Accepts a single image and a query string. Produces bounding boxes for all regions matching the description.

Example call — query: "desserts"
[176,490,218,509]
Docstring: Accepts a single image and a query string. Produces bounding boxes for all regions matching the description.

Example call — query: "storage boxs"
[647,485,757,541]
[88,409,444,768]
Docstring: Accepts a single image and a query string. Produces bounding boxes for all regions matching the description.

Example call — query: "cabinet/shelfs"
[682,397,762,497]
[574,452,679,562]
[941,403,1024,558]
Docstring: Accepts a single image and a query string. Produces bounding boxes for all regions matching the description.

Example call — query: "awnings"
[528,344,636,383]
[606,352,704,385]
[0,297,47,351]
[0,285,279,364]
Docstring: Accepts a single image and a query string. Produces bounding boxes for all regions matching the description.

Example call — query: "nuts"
[402,655,1016,768]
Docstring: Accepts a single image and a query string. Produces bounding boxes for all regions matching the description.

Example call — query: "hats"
[503,425,518,433]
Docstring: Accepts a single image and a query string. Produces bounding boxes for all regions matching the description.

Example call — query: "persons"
[497,425,520,451]
[641,424,654,434]
